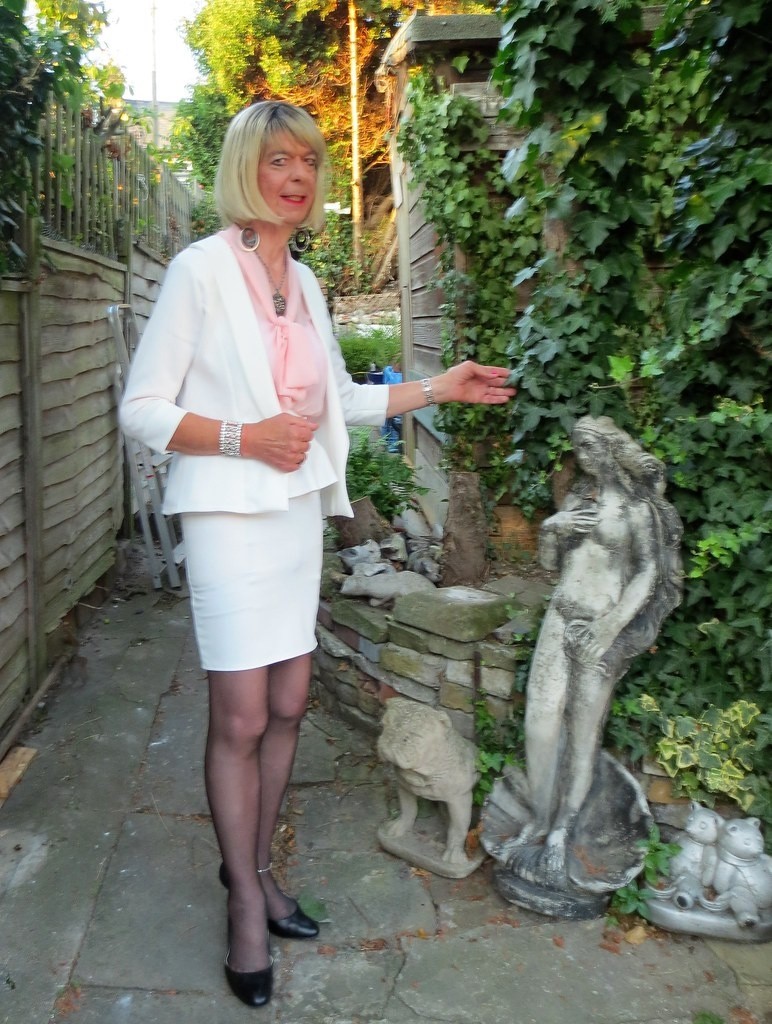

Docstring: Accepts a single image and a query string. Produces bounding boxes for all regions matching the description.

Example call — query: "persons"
[499,415,686,880]
[114,99,518,1008]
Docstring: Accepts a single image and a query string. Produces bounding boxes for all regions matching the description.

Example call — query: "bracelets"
[220,418,243,458]
[420,379,435,406]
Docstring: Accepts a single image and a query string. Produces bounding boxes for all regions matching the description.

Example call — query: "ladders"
[106,303,186,591]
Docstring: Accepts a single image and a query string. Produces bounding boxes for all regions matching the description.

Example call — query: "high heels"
[225,930,273,1007]
[219,862,319,939]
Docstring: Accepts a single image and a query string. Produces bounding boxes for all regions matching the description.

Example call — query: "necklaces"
[255,248,289,315]
